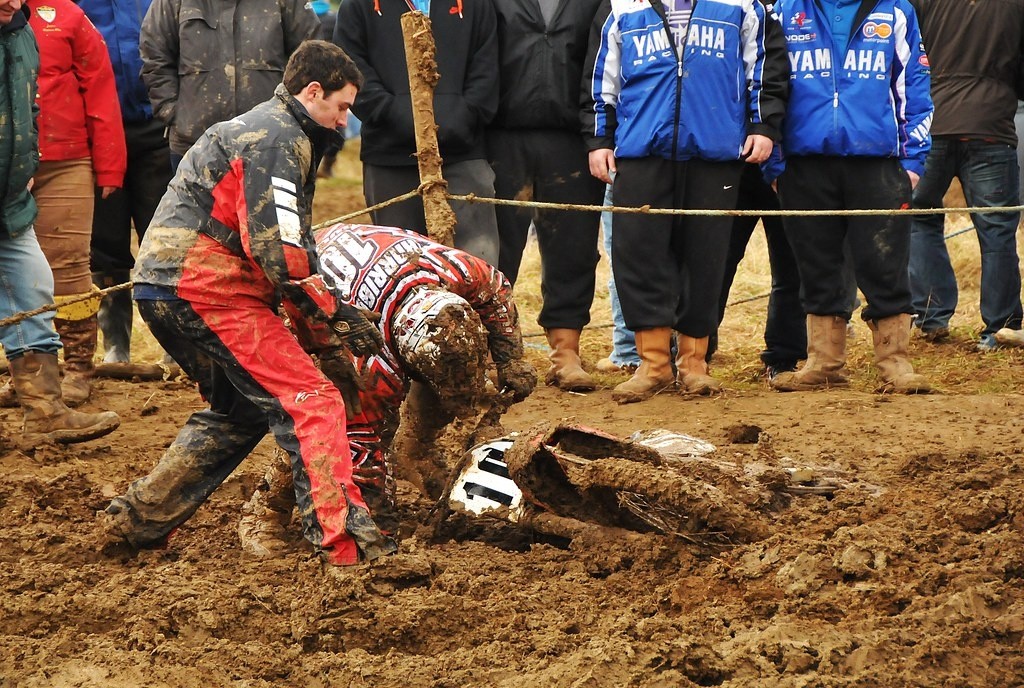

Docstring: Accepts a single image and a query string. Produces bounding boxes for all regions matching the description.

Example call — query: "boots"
[867,314,931,391]
[0,371,19,409]
[612,326,675,402]
[545,329,597,390]
[90,270,133,362]
[676,330,720,394]
[53,313,98,407]
[7,354,121,451]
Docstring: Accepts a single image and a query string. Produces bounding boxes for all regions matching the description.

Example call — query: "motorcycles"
[419,385,774,547]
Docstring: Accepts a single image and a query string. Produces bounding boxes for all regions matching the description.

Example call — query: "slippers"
[770,314,849,390]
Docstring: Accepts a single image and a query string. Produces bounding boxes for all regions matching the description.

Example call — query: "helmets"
[392,287,487,389]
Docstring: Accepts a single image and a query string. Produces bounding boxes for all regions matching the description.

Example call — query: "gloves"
[321,351,364,420]
[329,304,385,358]
[498,360,539,403]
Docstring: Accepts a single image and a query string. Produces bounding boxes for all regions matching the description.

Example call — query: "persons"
[99,39,537,565]
[0,0,1023,451]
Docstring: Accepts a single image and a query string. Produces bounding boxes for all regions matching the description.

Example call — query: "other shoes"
[976,334,1015,350]
[598,358,638,371]
[767,365,795,383]
[995,328,1024,346]
